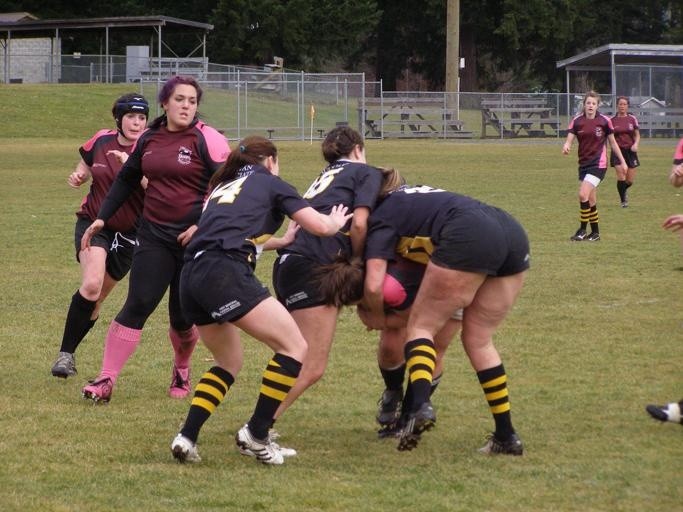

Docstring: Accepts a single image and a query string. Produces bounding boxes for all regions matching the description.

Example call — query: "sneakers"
[167,365,193,400]
[80,377,113,404]
[234,422,298,465]
[375,386,437,453]
[568,228,601,242]
[643,399,683,426]
[475,430,523,458]
[50,350,79,379]
[169,432,202,466]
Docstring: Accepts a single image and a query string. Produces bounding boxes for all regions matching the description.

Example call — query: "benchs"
[126,56,209,85]
[598,98,683,139]
[357,96,472,140]
[480,97,567,138]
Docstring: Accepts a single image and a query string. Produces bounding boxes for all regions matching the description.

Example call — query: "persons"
[669,138,683,188]
[608,94,640,208]
[50,92,151,379]
[561,91,628,242]
[168,134,354,466]
[81,75,232,403]
[645,212,683,424]
